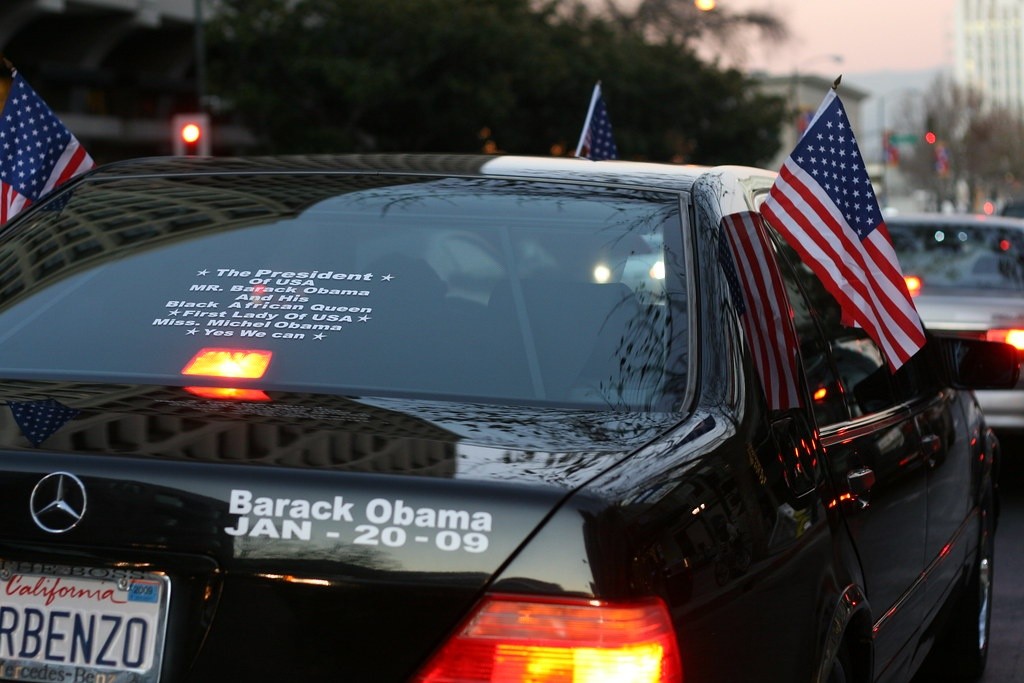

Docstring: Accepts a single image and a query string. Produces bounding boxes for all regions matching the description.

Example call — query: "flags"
[0,69,98,225]
[717,210,805,409]
[575,84,617,160]
[759,87,926,373]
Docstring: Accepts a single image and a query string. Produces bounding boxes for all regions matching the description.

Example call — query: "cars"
[1,153,1024,683]
[881,214,1024,432]
